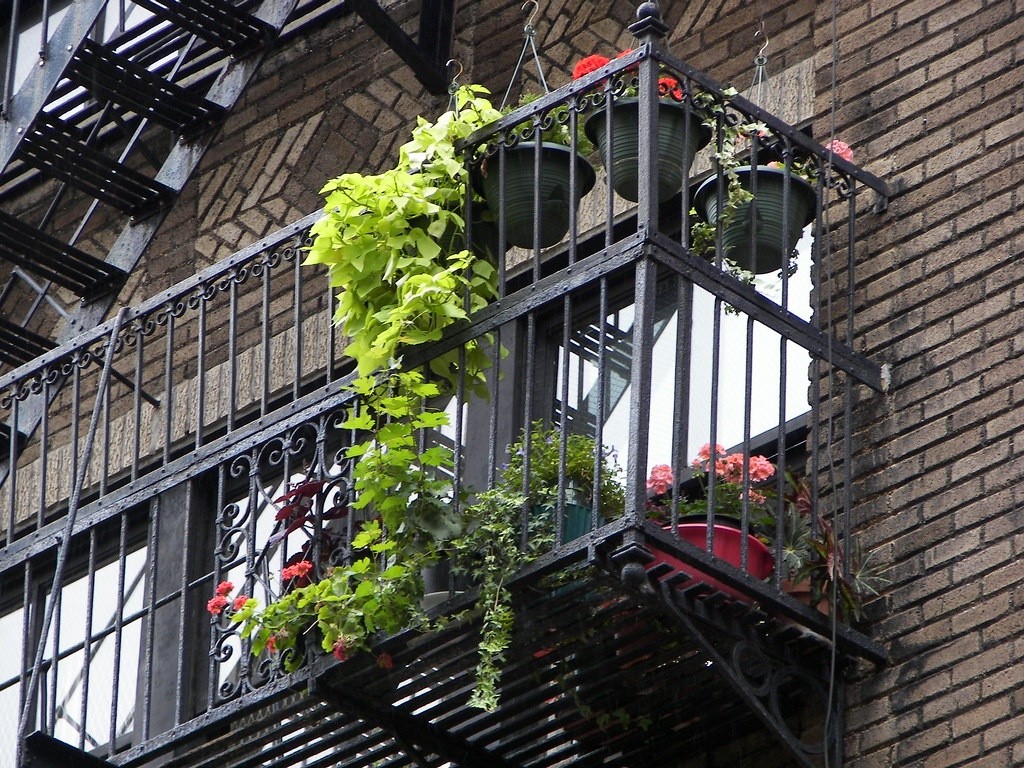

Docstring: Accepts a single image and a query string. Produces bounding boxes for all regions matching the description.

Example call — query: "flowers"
[737,129,854,183]
[632,445,775,530]
[573,49,756,315]
[207,479,394,669]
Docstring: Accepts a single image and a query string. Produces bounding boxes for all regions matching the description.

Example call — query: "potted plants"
[234,84,508,713]
[471,89,596,249]
[763,467,893,625]
[444,419,693,746]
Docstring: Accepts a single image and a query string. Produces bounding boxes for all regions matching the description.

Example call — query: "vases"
[694,166,817,274]
[644,510,774,617]
[584,96,713,203]
